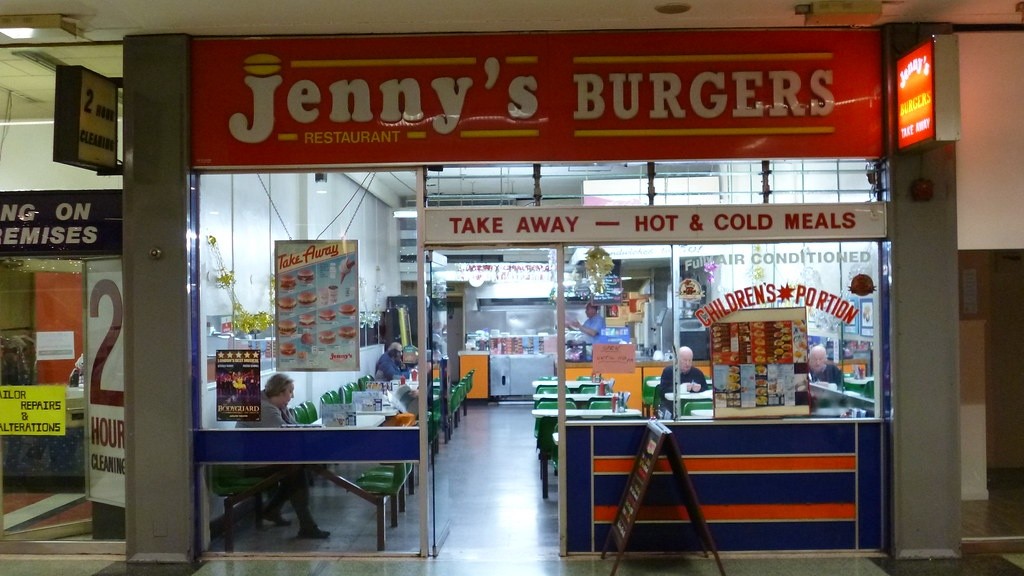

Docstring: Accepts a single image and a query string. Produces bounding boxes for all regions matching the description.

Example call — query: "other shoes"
[262,510,292,526]
[297,524,330,539]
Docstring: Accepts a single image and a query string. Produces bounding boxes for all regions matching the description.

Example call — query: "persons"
[375,342,409,382]
[808,345,845,392]
[235,374,330,539]
[565,302,606,344]
[660,346,709,419]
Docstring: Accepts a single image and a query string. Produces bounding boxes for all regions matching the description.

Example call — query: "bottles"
[635,350,641,363]
[595,385,600,395]
[618,391,626,412]
[595,373,599,382]
[593,375,595,383]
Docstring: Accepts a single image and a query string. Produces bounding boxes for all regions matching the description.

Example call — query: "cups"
[400,374,407,385]
[410,369,418,381]
[679,384,686,391]
[612,396,618,412]
[600,383,606,396]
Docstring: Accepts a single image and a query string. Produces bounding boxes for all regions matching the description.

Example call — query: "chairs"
[843,372,873,398]
[203,369,713,551]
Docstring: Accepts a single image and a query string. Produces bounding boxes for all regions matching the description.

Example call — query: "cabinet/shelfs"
[489,355,555,406]
[457,350,490,402]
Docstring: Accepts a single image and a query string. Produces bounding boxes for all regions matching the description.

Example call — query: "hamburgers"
[278,270,355,355]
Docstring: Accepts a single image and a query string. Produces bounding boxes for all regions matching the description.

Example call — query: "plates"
[679,392,690,395]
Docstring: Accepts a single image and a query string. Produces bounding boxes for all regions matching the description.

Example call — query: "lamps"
[391,207,417,217]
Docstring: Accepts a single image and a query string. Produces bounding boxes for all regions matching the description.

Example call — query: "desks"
[691,406,848,420]
[309,415,385,426]
[646,379,712,388]
[356,405,398,417]
[844,377,874,385]
[532,394,618,403]
[532,380,608,389]
[530,409,642,419]
[665,390,713,402]
[390,378,446,386]
